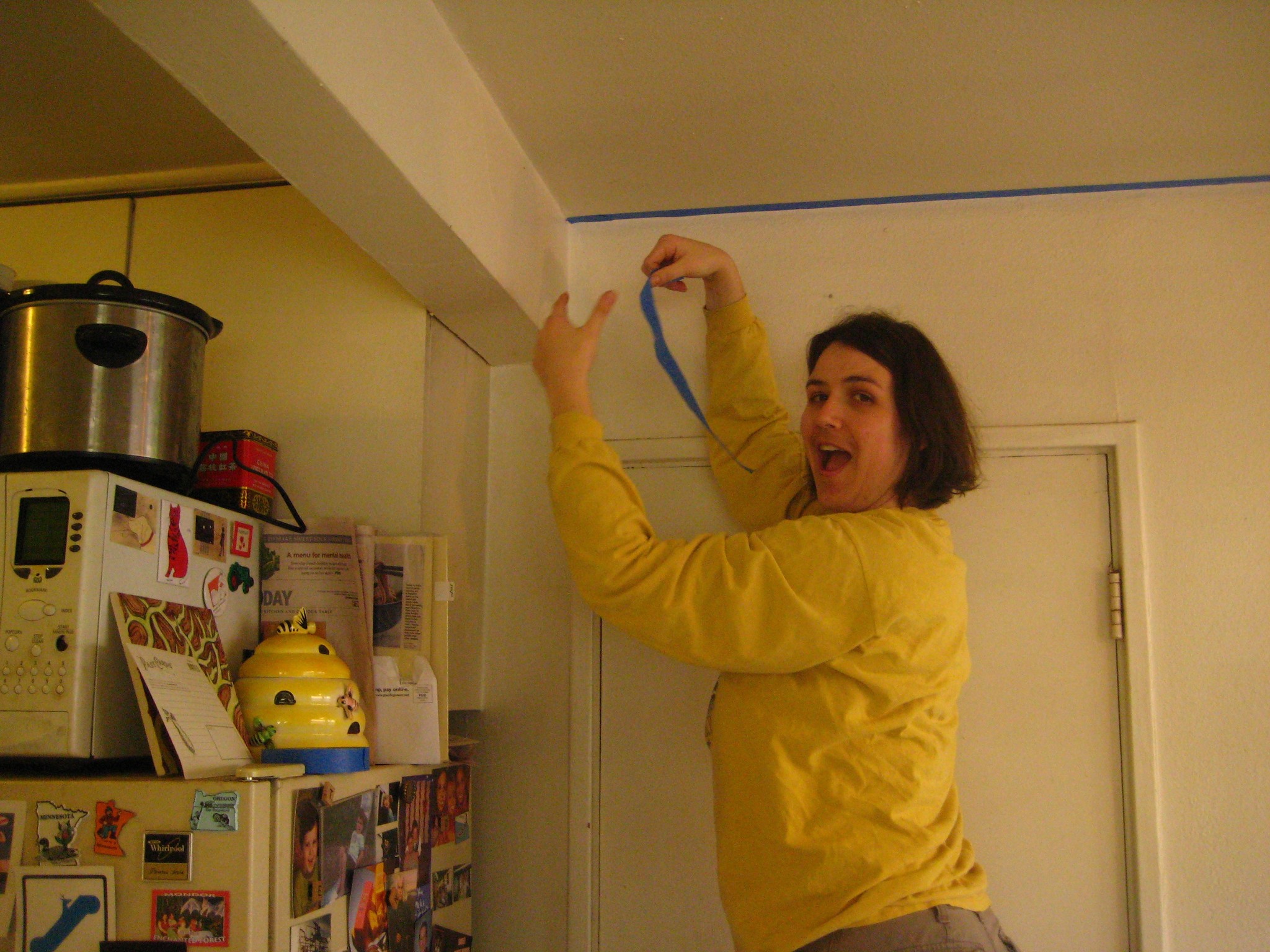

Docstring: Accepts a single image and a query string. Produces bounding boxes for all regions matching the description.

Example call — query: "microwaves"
[0,469,259,771]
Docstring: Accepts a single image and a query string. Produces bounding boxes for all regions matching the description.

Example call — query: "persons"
[293,765,472,952]
[157,910,225,942]
[533,233,1019,952]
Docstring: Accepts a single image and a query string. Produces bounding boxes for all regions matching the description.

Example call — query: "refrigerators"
[0,750,479,952]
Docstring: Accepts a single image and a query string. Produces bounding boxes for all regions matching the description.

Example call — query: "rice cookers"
[0,269,224,496]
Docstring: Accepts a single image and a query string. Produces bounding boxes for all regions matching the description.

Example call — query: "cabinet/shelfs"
[0,189,489,717]
